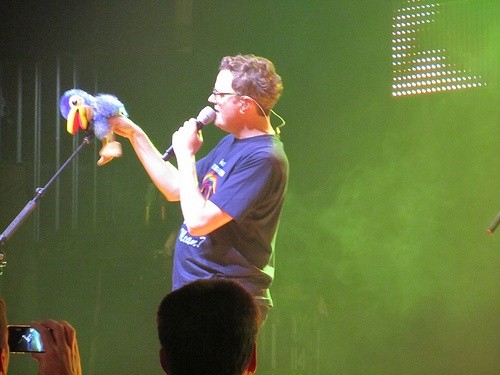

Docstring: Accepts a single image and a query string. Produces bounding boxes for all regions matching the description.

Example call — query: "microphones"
[84,131,95,144]
[162,106,216,161]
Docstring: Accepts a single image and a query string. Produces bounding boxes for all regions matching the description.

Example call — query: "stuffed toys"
[60,88,129,166]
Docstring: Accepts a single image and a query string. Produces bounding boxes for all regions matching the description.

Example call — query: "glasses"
[212,90,245,101]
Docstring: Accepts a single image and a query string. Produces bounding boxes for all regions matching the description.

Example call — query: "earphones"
[241,99,246,105]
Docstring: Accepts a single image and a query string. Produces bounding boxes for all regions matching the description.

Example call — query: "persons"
[157,276,263,375]
[0,299,83,375]
[107,54,290,336]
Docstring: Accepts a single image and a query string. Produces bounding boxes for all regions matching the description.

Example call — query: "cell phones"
[7,324,53,355]
